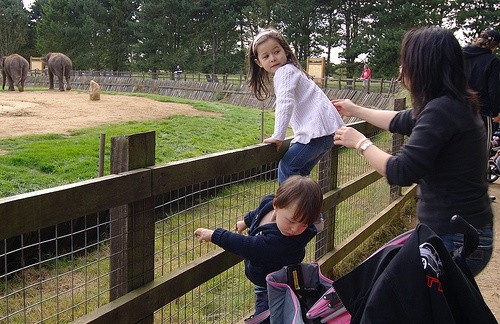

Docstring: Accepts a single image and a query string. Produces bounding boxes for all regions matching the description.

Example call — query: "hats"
[480,29,500,49]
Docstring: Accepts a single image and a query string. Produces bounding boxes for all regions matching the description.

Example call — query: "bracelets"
[355,138,369,149]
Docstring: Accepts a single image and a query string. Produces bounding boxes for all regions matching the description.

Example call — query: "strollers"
[486,115,500,182]
[266,213,500,324]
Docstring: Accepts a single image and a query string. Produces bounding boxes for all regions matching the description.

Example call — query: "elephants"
[42,52,72,91]
[0,54,29,92]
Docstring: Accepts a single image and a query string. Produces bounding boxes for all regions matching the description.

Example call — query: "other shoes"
[314,218,325,232]
[488,196,496,200]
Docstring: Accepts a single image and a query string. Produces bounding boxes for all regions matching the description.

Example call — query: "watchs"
[359,140,374,157]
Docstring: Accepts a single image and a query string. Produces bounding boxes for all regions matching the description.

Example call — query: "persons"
[360,64,371,94]
[194,176,325,324]
[330,27,500,277]
[249,27,346,235]
[174,65,183,80]
